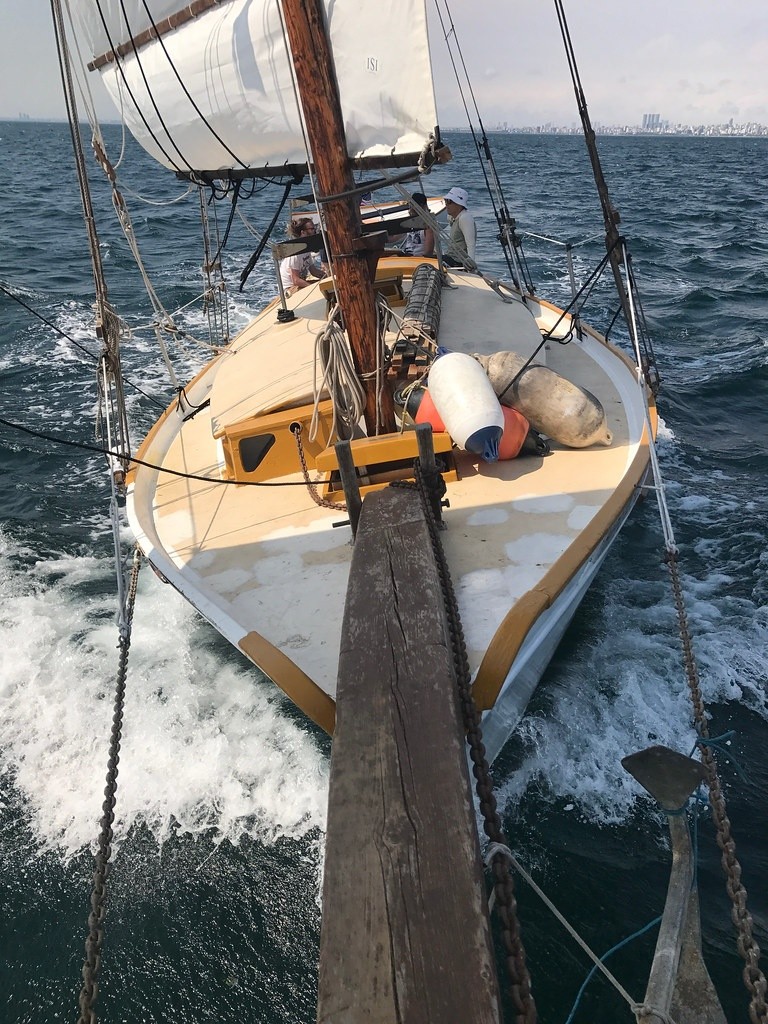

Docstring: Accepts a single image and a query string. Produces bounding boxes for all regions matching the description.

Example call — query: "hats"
[406,192,427,208]
[443,186,470,211]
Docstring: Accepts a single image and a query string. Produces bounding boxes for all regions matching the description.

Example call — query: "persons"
[277,218,328,300]
[386,193,435,258]
[319,207,335,277]
[435,186,477,271]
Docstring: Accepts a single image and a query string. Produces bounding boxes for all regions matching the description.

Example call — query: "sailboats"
[0,1,768,1022]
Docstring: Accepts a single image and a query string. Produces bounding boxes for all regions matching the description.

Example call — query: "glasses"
[444,198,454,204]
[304,224,317,232]
